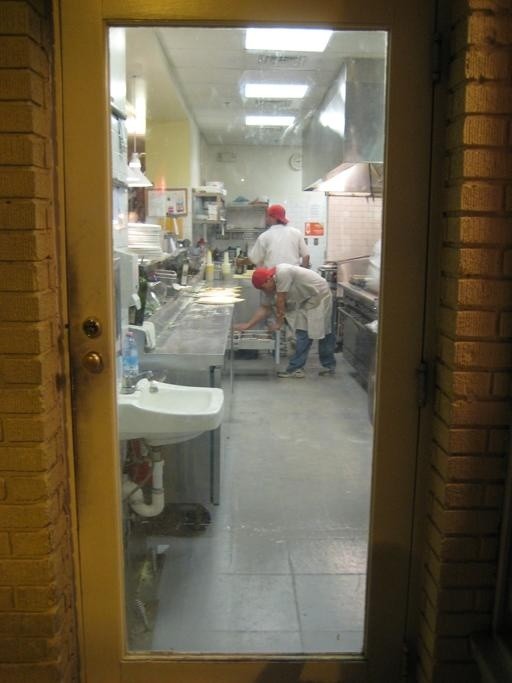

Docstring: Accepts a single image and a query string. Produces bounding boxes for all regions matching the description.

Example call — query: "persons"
[233,263,335,379]
[237,204,311,269]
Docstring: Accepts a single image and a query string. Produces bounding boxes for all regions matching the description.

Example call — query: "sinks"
[119,379,225,445]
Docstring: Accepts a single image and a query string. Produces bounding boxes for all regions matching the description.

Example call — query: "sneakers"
[276,365,305,379]
[318,362,337,377]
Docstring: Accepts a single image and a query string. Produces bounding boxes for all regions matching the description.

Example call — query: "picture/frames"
[146,187,188,217]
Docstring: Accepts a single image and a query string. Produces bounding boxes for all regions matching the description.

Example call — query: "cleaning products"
[121,331,139,378]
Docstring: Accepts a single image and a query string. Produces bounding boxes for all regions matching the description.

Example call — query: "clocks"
[289,152,302,170]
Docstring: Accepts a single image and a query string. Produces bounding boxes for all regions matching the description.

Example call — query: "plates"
[127,220,163,259]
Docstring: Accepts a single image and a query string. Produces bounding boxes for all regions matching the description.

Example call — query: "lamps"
[122,72,154,188]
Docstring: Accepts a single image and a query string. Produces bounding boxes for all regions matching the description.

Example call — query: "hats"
[266,204,290,225]
[252,266,277,288]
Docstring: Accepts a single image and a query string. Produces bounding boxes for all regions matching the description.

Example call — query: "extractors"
[299,62,383,199]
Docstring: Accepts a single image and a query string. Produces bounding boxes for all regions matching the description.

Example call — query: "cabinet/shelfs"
[192,190,268,233]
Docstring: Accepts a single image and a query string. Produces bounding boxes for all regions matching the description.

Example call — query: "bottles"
[123,329,139,379]
[237,249,245,273]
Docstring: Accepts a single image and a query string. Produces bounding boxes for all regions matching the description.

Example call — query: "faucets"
[121,370,160,395]
